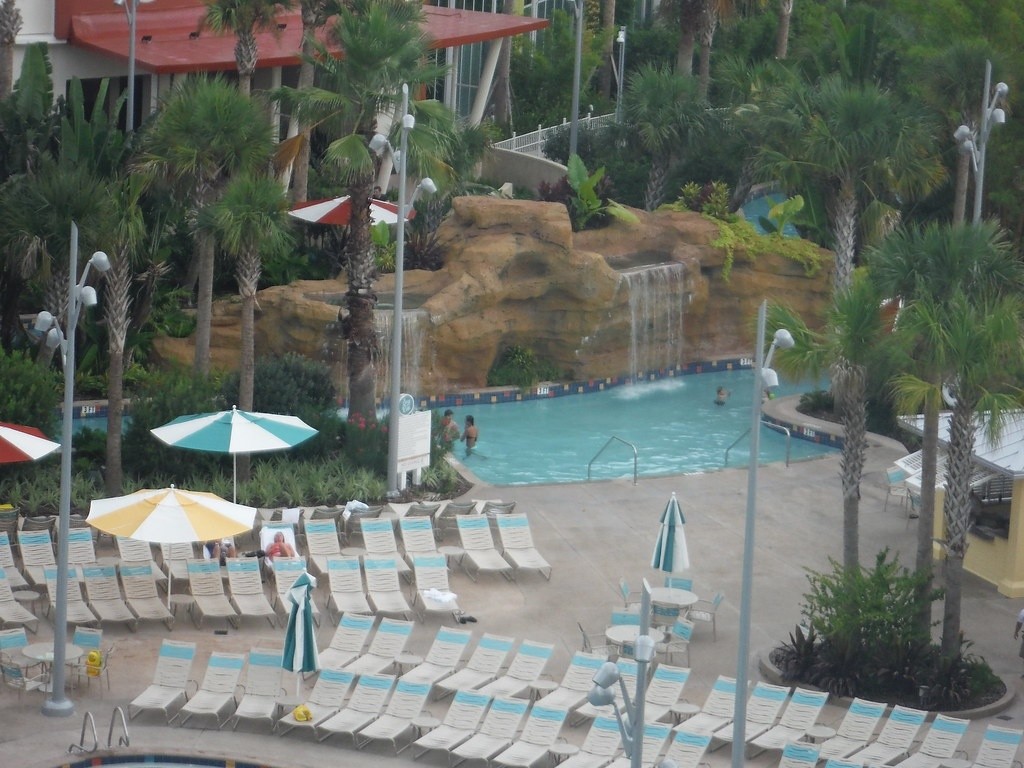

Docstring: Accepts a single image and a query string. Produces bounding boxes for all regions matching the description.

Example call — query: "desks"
[410,716,442,737]
[276,695,305,715]
[237,550,264,559]
[604,622,666,657]
[528,679,559,701]
[438,546,466,569]
[22,642,83,692]
[671,703,701,723]
[14,590,41,615]
[167,594,195,624]
[806,726,836,744]
[942,759,973,768]
[341,548,366,555]
[647,585,699,622]
[548,743,579,764]
[97,557,120,565]
[394,654,423,677]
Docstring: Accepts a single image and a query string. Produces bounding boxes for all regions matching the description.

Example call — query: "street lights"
[955,56,1008,226]
[32,226,111,714]
[731,294,795,768]
[370,85,439,495]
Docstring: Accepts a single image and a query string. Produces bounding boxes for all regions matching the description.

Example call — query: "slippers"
[460,616,477,624]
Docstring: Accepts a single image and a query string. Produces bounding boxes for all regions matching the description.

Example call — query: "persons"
[0,492,15,511]
[371,186,387,201]
[202,533,298,558]
[714,386,731,408]
[440,410,486,461]
[762,388,776,403]
[1013,609,1024,684]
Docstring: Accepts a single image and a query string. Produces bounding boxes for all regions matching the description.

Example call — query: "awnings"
[78,4,550,75]
[894,445,999,492]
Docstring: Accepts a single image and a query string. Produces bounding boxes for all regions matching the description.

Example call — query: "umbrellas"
[149,405,319,502]
[651,490,690,589]
[287,192,416,226]
[0,422,61,465]
[281,567,320,702]
[85,483,257,624]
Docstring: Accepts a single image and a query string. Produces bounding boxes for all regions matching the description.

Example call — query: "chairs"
[0,497,1024,768]
[883,465,908,513]
